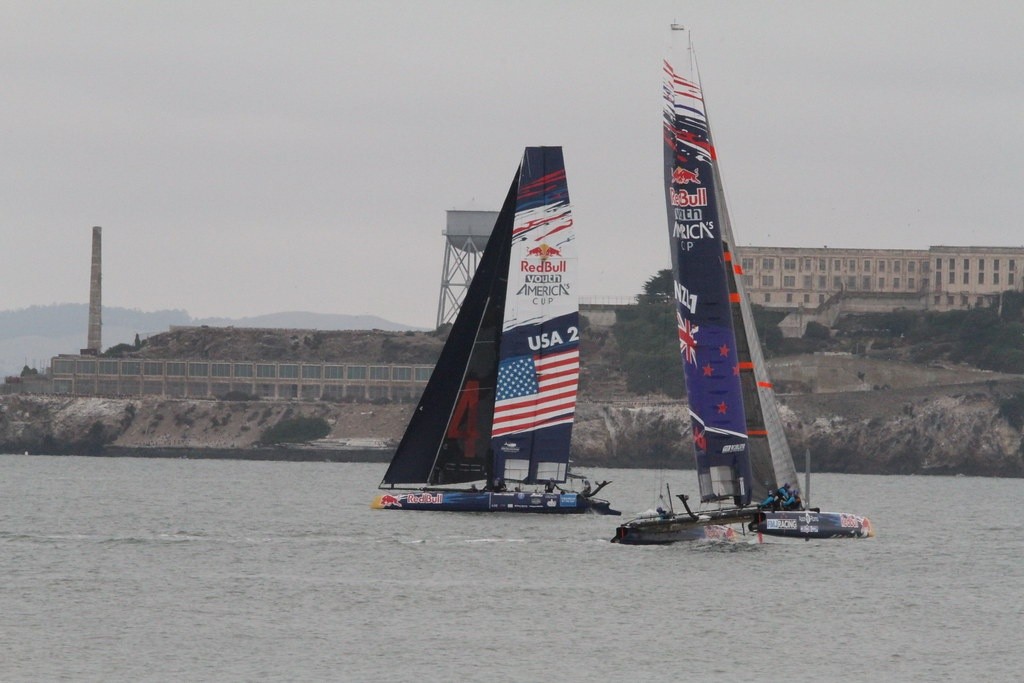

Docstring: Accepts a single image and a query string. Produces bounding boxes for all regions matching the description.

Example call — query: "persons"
[470,484,476,489]
[544,478,563,493]
[584,481,591,492]
[761,482,802,510]
[656,507,667,519]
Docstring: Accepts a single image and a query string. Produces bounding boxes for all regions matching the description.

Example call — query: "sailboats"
[609,20,877,546]
[369,144,623,516]
